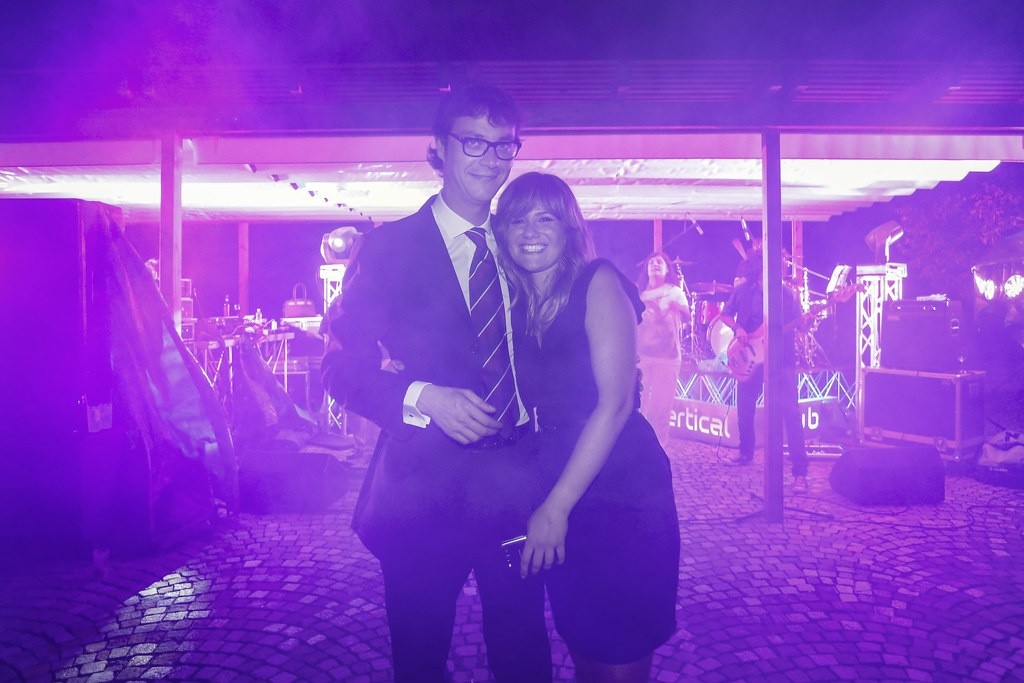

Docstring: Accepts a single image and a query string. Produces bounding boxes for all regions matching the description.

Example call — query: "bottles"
[223,294,230,317]
[256,308,263,322]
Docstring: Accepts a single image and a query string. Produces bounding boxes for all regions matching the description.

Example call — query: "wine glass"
[234,303,241,317]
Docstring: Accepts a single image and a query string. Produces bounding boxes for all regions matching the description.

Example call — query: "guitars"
[727,279,865,384]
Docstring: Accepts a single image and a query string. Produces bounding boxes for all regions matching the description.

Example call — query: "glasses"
[439,130,523,160]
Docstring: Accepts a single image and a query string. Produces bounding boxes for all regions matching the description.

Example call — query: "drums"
[680,315,737,360]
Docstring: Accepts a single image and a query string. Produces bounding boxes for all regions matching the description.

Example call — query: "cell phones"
[500,534,563,578]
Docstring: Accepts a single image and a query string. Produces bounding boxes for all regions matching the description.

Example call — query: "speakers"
[235,450,347,516]
[825,444,946,509]
[878,296,968,375]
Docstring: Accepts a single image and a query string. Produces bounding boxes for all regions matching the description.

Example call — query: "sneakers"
[722,453,752,466]
[793,475,809,493]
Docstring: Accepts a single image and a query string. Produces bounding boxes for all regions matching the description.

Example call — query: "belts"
[456,422,531,451]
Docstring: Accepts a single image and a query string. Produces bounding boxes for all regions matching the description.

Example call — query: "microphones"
[686,212,703,235]
[740,217,750,241]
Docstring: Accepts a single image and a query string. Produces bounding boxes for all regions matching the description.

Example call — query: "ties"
[463,225,521,439]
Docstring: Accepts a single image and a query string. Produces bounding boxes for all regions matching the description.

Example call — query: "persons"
[314,81,645,683]
[716,250,820,492]
[382,168,683,683]
[634,253,691,448]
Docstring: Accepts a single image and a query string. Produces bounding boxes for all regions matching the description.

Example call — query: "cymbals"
[688,281,733,294]
[667,258,696,268]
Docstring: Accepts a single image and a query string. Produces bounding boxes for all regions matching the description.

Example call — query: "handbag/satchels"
[977,430,1024,469]
[283,282,316,318]
